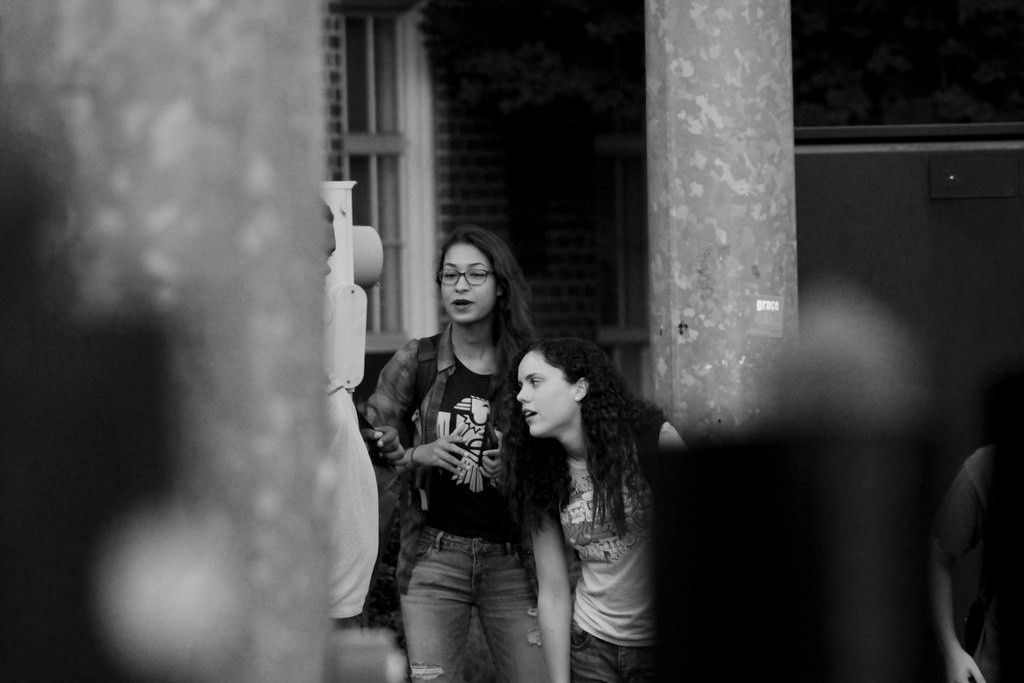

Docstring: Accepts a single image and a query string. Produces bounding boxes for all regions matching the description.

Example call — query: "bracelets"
[401,445,419,471]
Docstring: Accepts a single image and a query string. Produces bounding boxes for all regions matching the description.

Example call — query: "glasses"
[437,269,496,285]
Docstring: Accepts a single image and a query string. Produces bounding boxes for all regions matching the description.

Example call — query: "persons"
[320,195,341,295]
[519,333,699,681]
[920,373,1023,683]
[364,224,553,683]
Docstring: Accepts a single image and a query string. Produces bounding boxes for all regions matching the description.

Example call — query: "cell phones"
[478,423,498,485]
